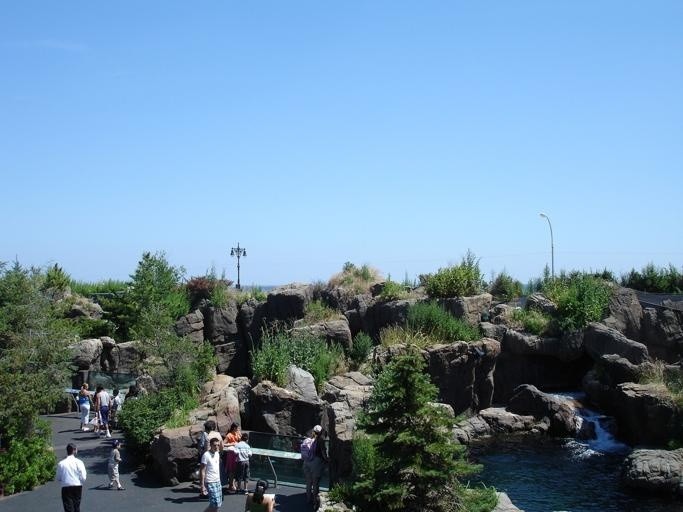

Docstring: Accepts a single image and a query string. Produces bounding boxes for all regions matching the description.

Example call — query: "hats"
[314,425,322,432]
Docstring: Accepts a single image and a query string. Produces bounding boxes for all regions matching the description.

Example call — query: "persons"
[107,439,126,490]
[197,420,253,501]
[245,478,276,512]
[77,382,139,439]
[54,443,87,511]
[303,425,332,511]
[200,438,223,512]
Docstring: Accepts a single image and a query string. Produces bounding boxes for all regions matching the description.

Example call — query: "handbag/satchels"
[223,444,234,451]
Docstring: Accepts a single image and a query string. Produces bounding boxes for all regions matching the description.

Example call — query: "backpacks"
[301,438,317,461]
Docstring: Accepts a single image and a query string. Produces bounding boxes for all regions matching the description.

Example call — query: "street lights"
[229,242,245,284]
[538,213,554,282]
